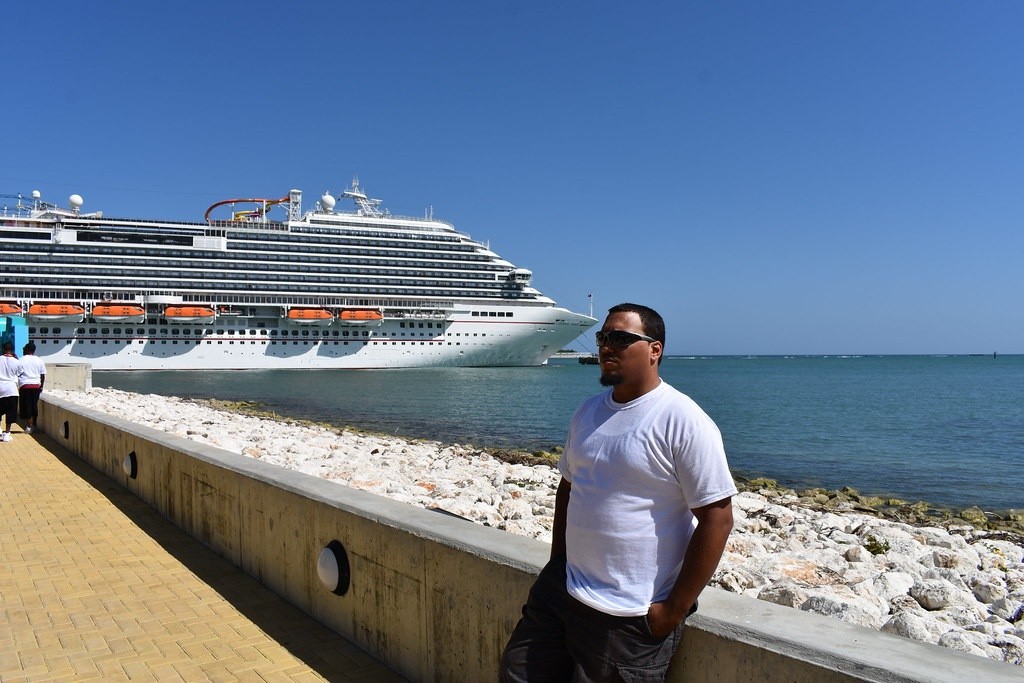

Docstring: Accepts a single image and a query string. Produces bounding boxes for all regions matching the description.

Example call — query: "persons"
[499,303,739,683]
[18,342,46,434]
[0,342,22,442]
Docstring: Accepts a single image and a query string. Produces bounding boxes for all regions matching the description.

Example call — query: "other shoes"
[0,432,3,441]
[24,426,35,434]
[2,431,12,442]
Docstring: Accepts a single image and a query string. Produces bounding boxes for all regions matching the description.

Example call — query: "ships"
[1,170,599,372]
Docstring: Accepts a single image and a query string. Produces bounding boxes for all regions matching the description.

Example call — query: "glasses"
[595,329,658,350]
